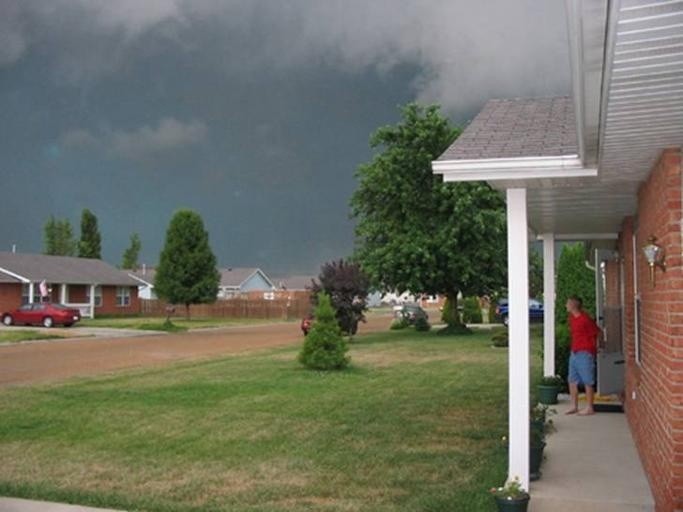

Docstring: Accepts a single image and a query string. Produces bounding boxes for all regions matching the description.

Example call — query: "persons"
[565,295,602,415]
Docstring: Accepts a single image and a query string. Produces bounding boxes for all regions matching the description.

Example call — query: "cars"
[301,310,358,335]
[0,302,80,327]
[397,305,428,324]
[495,298,543,327]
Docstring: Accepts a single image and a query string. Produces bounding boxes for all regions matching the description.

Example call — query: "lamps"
[641,235,666,289]
